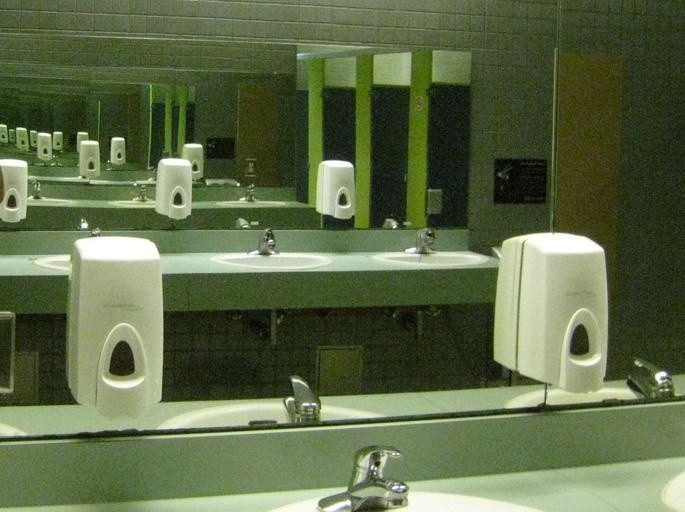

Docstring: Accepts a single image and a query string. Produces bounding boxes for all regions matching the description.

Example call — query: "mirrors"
[0,28,685,443]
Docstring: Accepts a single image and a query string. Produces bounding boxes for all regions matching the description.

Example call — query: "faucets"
[627,356,675,401]
[283,373,323,424]
[133,184,153,202]
[317,443,410,511]
[31,177,42,199]
[76,216,101,237]
[239,182,280,255]
[382,217,436,254]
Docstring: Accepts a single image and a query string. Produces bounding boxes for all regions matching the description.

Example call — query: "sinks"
[267,493,542,512]
[26,197,286,207]
[504,388,642,410]
[34,254,72,271]
[660,470,685,511]
[208,251,490,270]
[0,422,28,437]
[155,402,387,430]
